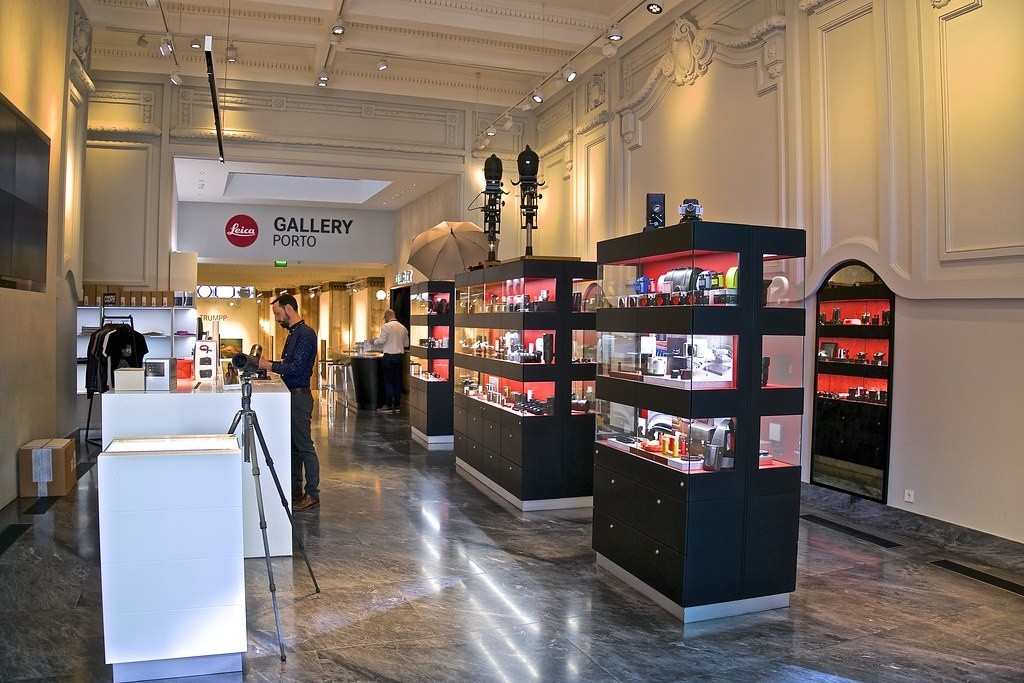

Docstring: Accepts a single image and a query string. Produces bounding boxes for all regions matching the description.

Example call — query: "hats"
[383,309,395,320]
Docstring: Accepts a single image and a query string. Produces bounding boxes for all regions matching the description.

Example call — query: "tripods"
[228,374,321,661]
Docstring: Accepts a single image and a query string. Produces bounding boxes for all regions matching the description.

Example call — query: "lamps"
[607,23,623,41]
[559,66,577,83]
[317,70,329,88]
[226,280,364,306]
[169,66,182,85]
[160,33,172,58]
[475,113,513,150]
[646,0,664,15]
[376,56,389,71]
[137,34,147,47]
[532,86,543,103]
[225,41,237,62]
[331,15,345,36]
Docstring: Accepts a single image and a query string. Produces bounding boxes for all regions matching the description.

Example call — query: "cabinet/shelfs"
[76,306,197,429]
[409,280,455,437]
[455,260,596,501]
[591,220,807,604]
[811,284,894,470]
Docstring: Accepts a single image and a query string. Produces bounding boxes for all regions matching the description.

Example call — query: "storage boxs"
[18,438,76,496]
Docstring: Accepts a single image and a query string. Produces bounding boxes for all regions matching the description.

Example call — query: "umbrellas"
[407,220,500,282]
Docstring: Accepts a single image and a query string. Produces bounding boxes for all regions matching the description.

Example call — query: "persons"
[369,308,410,415]
[224,362,238,385]
[259,295,321,511]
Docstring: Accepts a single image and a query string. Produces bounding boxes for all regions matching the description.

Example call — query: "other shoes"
[377,405,394,415]
[395,408,400,414]
[293,491,320,512]
[293,489,304,499]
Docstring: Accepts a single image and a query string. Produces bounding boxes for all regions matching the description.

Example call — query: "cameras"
[645,193,665,228]
[678,202,703,218]
[519,350,542,363]
